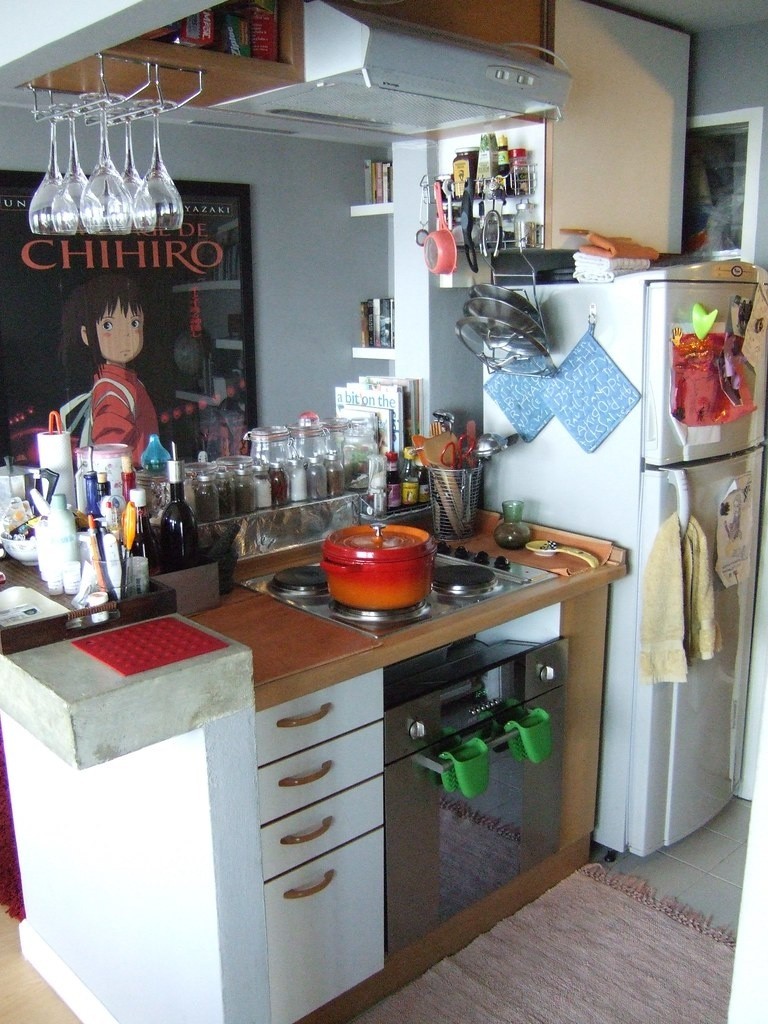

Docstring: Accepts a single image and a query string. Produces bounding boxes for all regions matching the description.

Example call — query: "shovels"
[412,433,463,536]
[421,431,464,519]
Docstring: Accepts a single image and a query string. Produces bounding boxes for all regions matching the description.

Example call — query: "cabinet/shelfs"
[546,0,690,257]
[350,202,394,360]
[172,217,244,408]
[252,667,387,1007]
[29,1,304,107]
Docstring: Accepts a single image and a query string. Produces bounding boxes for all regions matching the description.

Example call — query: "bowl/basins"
[0,532,39,566]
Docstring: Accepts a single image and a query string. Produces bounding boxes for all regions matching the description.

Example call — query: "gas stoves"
[236,537,560,639]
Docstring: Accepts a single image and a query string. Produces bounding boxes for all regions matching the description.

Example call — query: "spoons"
[526,541,599,568]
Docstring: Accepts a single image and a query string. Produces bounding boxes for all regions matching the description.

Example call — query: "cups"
[90,555,132,601]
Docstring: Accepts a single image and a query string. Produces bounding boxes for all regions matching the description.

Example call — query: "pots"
[320,521,438,608]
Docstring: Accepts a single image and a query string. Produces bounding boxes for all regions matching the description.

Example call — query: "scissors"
[440,434,476,478]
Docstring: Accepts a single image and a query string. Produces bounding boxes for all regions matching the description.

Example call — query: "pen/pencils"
[88,515,105,591]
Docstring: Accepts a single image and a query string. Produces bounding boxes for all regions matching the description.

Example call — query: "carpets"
[340,862,736,1023]
[434,797,521,912]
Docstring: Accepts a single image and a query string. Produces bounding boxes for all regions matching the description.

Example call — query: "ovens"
[382,634,562,952]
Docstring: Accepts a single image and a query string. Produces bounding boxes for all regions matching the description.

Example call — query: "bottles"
[418,465,430,504]
[38,410,385,596]
[434,175,453,202]
[514,203,537,248]
[453,147,479,200]
[493,500,530,549]
[496,135,514,196]
[508,148,534,196]
[87,591,109,623]
[386,452,401,511]
[401,446,419,507]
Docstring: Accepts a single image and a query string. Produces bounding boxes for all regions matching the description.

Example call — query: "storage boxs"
[156,548,220,615]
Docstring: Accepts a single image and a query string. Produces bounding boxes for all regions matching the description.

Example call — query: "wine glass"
[28,92,183,236]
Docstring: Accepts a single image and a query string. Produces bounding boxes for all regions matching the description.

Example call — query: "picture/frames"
[0,169,260,467]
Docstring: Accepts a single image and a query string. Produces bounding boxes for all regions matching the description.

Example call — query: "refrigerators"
[481,263,766,859]
